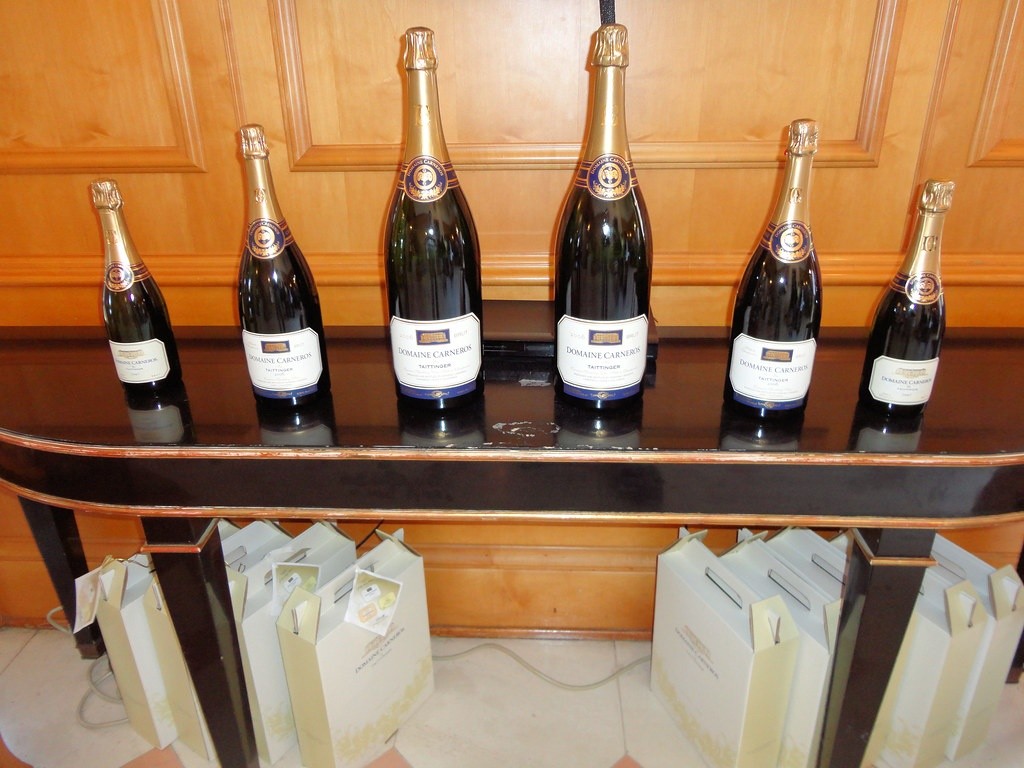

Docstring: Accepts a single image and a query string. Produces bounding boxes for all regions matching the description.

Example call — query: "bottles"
[724,120,823,430]
[860,181,955,413]
[237,122,331,409]
[396,394,487,449]
[123,381,194,448]
[553,24,655,412]
[383,27,487,413]
[847,400,924,453]
[554,394,643,448]
[90,180,182,396]
[718,400,804,454]
[253,393,337,447]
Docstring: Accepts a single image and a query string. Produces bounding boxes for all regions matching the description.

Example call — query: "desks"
[0,327,1024,768]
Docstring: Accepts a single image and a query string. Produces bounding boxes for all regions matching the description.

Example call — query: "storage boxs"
[72,518,436,768]
[649,526,1024,768]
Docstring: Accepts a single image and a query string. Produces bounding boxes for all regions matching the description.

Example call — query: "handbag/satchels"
[716,527,840,768]
[275,530,435,768]
[766,524,917,768]
[832,529,988,768]
[929,532,1024,768]
[146,518,295,768]
[74,517,239,751]
[230,517,355,768]
[648,526,797,768]
